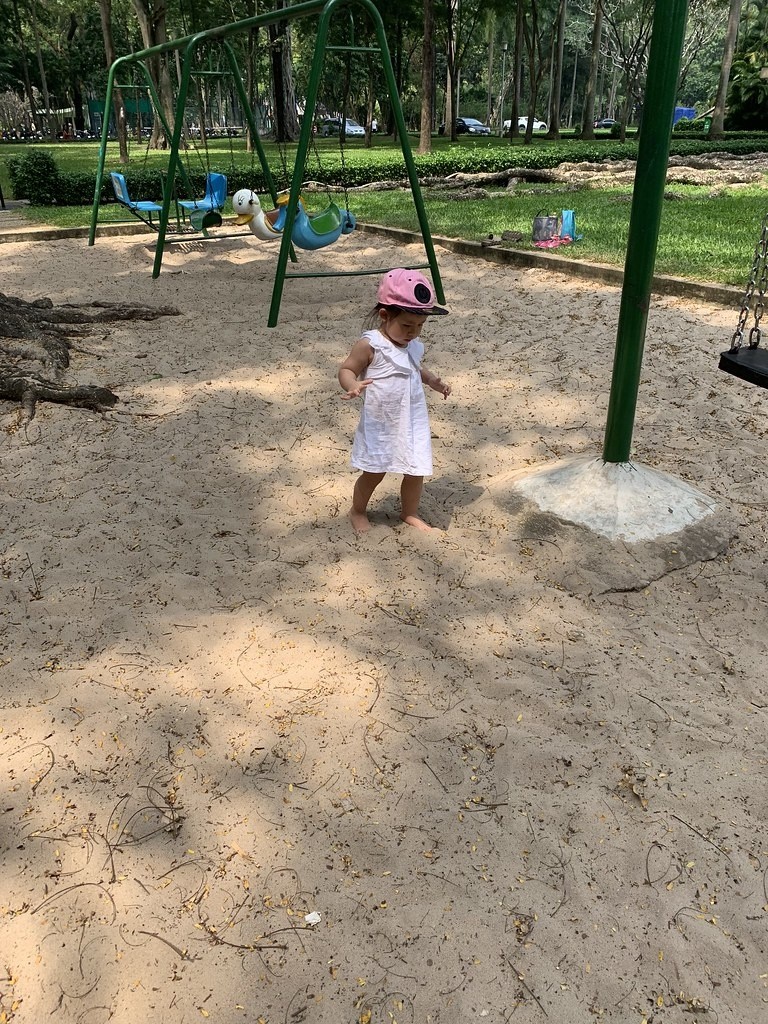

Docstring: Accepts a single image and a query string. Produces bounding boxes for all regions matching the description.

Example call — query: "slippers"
[501,230,523,241]
[481,233,501,246]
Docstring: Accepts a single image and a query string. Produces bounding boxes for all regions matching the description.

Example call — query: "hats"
[377,268,449,315]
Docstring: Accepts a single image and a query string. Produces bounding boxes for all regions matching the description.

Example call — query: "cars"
[593,117,617,129]
[321,118,366,138]
[503,116,548,131]
[438,117,491,135]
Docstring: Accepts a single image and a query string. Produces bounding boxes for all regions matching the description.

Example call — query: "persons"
[338,267,453,535]
[594,121,598,128]
[372,117,379,134]
[189,126,248,139]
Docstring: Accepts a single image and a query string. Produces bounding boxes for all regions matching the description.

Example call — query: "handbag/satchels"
[561,210,575,241]
[532,209,558,240]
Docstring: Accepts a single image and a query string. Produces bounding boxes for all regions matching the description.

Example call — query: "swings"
[248,2,361,254]
[106,33,228,235]
[198,17,283,249]
